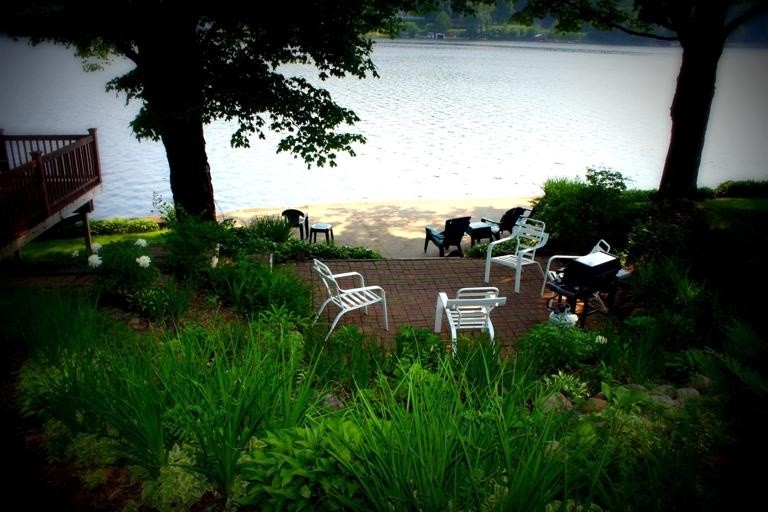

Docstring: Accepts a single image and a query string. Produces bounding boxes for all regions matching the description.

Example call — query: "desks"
[546,269,637,329]
[309,224,334,246]
[466,222,493,245]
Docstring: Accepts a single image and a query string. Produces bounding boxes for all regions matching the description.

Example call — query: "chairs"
[540,239,610,312]
[481,207,525,240]
[434,287,507,360]
[282,209,308,240]
[424,216,471,257]
[484,218,545,294]
[310,258,389,342]
